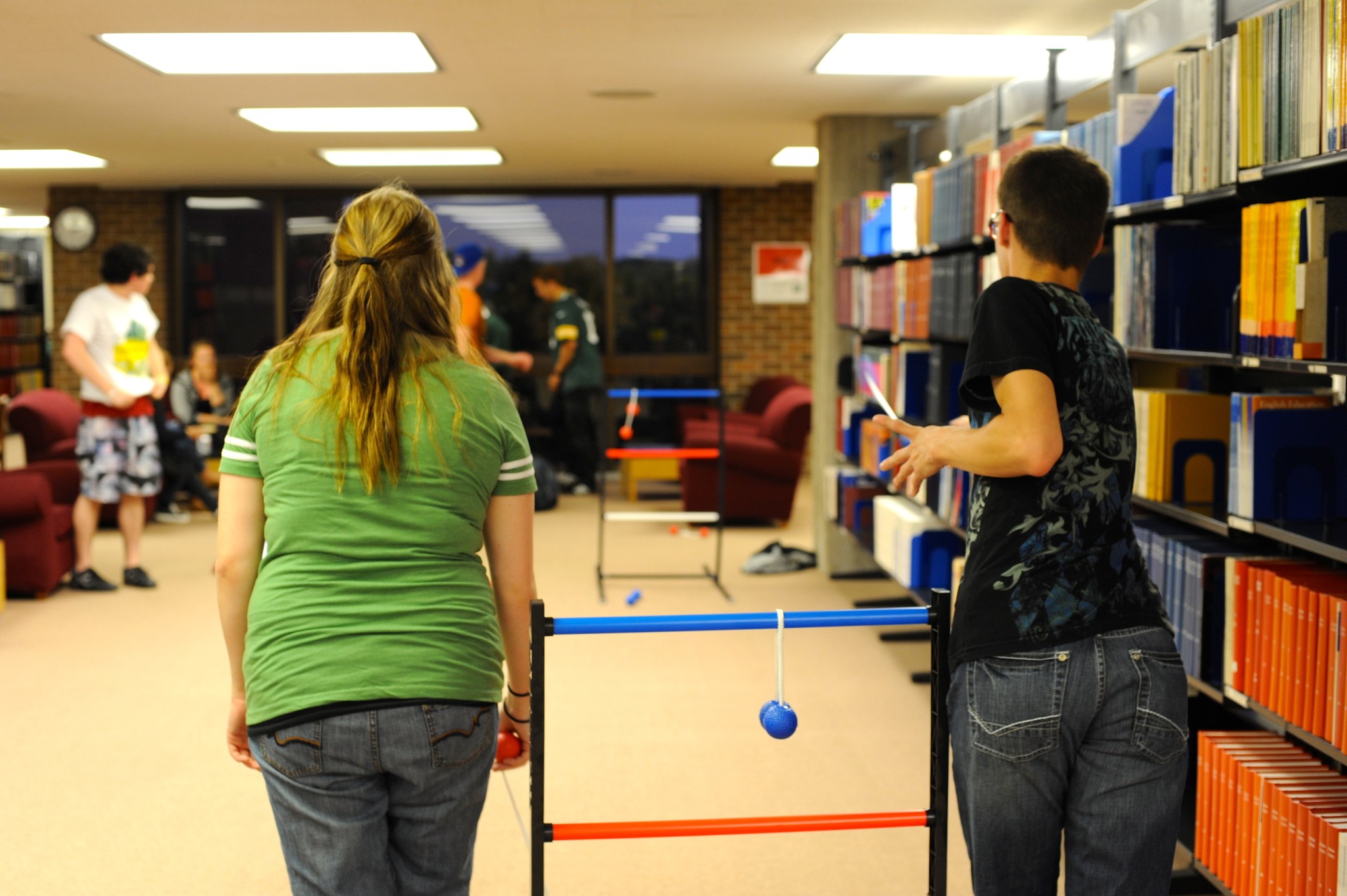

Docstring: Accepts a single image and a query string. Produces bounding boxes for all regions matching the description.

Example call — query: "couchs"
[680,374,813,528]
[0,388,154,601]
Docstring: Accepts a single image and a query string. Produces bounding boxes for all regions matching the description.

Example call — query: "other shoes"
[123,567,155,588]
[155,502,190,523]
[72,567,113,590]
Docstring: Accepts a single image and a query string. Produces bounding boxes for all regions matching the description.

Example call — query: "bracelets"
[553,371,561,375]
[508,679,532,697]
[503,698,530,723]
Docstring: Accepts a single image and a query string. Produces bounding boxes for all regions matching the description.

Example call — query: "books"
[836,0,1347,895]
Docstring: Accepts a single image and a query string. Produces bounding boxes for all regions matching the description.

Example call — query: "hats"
[453,240,481,275]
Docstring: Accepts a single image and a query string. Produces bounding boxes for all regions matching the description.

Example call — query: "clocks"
[52,204,99,253]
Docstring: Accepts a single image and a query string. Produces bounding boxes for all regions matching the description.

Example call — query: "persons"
[530,264,603,496]
[58,242,170,590]
[873,142,1185,896]
[170,340,238,463]
[149,348,219,523]
[447,242,534,372]
[214,179,538,896]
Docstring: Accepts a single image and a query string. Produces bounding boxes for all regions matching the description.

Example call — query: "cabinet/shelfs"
[830,150,1347,896]
